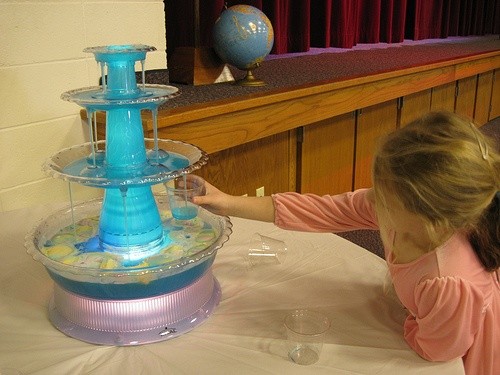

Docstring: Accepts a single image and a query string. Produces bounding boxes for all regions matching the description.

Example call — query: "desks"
[0,189,470,375]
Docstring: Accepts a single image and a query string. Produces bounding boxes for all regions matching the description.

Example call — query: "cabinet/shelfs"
[173,68,500,196]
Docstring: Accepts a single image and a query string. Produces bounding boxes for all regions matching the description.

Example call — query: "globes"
[212,2,274,87]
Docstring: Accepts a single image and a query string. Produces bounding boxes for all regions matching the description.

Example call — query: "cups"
[163,175,205,220]
[248,233,287,264]
[283,309,332,365]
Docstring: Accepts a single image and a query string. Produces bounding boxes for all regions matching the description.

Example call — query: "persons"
[174,112,500,375]
